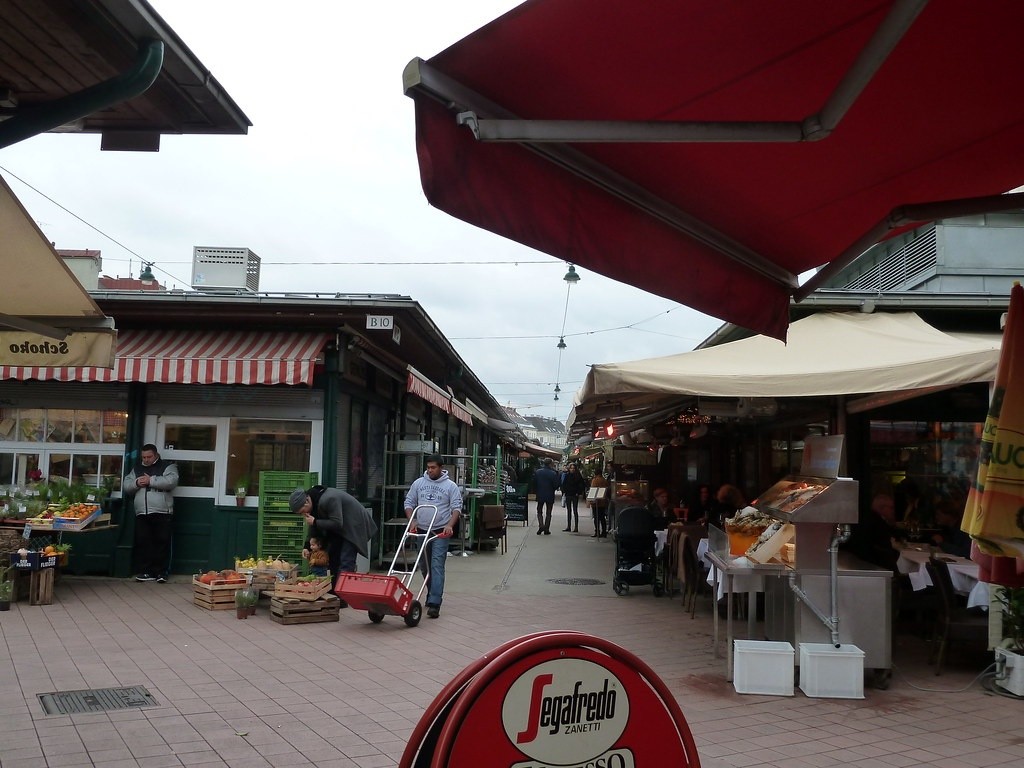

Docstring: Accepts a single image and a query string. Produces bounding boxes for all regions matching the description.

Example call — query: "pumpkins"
[44,546,64,555]
[198,569,247,585]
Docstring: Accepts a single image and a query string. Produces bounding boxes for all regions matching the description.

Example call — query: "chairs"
[669,528,710,619]
[474,505,508,555]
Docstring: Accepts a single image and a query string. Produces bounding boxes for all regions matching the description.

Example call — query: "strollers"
[612,507,668,597]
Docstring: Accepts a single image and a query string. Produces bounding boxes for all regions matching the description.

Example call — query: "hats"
[289,489,307,513]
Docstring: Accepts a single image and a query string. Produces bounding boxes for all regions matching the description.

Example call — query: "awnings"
[353,335,451,414]
[451,396,474,426]
[402,0,1024,346]
[564,310,1003,449]
[523,442,560,459]
[0,329,330,386]
[0,174,118,370]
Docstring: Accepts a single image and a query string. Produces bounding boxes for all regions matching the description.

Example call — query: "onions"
[290,581,323,594]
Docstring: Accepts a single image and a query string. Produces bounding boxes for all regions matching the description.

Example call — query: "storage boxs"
[734,640,795,696]
[0,478,115,611]
[192,471,413,625]
[798,643,865,698]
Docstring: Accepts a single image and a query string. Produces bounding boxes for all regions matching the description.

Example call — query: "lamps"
[563,265,581,285]
[138,263,156,286]
[592,420,599,438]
[553,384,561,426]
[556,337,567,350]
[602,417,614,438]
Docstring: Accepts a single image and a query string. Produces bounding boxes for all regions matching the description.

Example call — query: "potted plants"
[235,588,252,619]
[993,585,1024,697]
[245,594,258,615]
[231,477,251,506]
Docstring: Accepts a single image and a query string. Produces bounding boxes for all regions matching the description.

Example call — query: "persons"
[606,461,635,533]
[899,479,928,521]
[649,484,740,537]
[289,485,378,608]
[404,456,463,618]
[123,444,179,582]
[534,459,559,535]
[560,464,584,532]
[855,494,948,642]
[587,468,609,538]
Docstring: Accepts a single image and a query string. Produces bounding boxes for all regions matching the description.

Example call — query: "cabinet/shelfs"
[378,432,431,567]
[437,443,503,552]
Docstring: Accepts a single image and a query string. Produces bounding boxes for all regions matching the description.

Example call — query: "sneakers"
[136,572,156,581]
[156,573,168,582]
[427,605,440,618]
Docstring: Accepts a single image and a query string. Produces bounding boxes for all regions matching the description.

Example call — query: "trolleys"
[334,505,453,627]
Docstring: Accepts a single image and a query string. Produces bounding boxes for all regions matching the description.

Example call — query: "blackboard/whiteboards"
[504,483,529,521]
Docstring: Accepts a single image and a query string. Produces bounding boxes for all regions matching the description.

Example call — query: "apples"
[236,558,274,567]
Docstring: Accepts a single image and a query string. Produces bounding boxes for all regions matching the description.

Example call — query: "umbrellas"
[960,281,1024,557]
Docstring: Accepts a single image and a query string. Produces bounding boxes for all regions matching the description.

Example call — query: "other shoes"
[544,530,551,535]
[591,530,600,538]
[600,530,607,537]
[562,527,571,532]
[573,527,578,532]
[537,525,545,535]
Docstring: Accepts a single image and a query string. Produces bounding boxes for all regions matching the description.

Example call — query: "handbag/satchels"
[561,493,567,508]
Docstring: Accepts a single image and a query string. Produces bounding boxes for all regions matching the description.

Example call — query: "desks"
[668,521,709,551]
[897,543,987,676]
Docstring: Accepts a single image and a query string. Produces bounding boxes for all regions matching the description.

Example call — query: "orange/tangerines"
[62,504,98,520]
